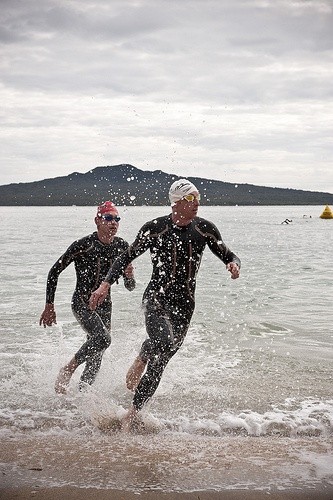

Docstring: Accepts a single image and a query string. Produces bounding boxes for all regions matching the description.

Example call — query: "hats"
[98,201,118,213]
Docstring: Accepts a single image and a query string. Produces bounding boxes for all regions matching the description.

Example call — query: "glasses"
[99,214,120,222]
[182,193,202,202]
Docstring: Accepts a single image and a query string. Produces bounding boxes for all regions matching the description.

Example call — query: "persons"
[89,179,241,431]
[39,200,135,395]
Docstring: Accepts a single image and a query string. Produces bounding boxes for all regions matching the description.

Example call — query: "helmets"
[168,179,199,203]
